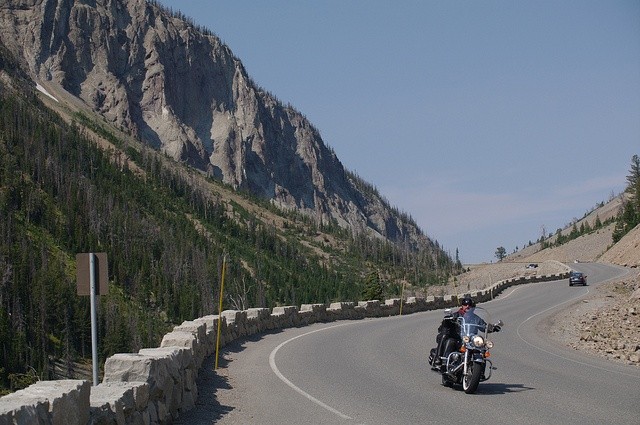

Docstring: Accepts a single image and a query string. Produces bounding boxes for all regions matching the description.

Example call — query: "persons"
[440,297,501,373]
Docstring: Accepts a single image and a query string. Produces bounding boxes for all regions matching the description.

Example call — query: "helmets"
[462,298,473,305]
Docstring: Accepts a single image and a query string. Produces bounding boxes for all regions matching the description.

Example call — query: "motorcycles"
[430,306,503,393]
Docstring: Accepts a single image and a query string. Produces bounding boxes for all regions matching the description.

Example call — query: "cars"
[569,271,588,285]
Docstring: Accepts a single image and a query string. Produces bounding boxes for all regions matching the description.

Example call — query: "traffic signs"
[76,251,109,382]
